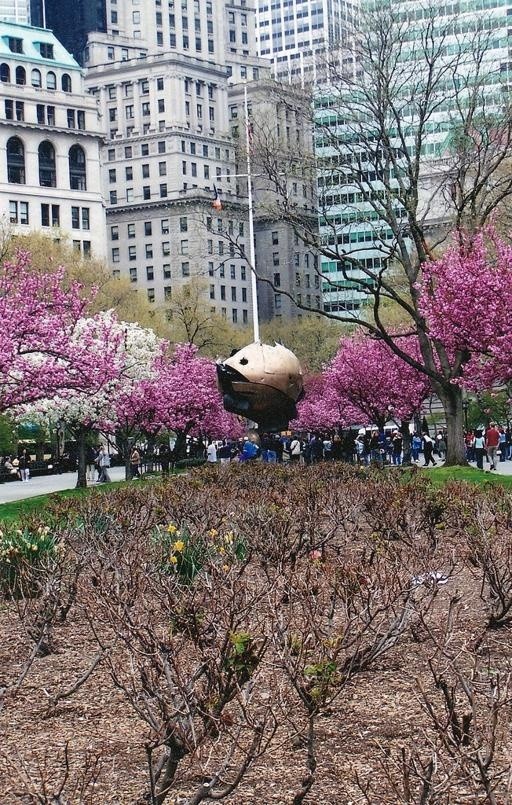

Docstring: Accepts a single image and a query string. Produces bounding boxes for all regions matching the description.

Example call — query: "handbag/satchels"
[291,446,296,451]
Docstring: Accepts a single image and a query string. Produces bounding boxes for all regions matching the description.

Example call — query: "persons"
[86,445,111,482]
[153,446,160,472]
[4,448,31,483]
[131,448,141,477]
[204,422,512,472]
[159,443,170,478]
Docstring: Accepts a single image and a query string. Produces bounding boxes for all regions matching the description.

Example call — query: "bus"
[9,439,78,463]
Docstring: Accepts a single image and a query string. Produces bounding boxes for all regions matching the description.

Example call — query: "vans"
[355,423,415,444]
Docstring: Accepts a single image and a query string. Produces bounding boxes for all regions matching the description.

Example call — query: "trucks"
[170,434,223,454]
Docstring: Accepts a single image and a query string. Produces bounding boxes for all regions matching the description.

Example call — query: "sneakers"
[489,465,496,470]
[432,462,437,466]
[422,463,428,466]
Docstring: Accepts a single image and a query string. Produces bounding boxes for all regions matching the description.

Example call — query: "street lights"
[209,172,292,342]
[462,399,470,435]
